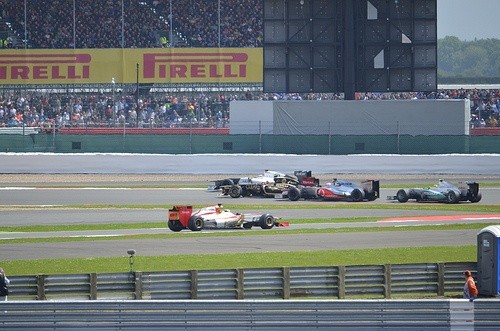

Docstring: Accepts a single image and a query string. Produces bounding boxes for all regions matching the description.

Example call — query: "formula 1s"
[285,178,380,203]
[170,202,288,235]
[212,168,301,199]
[397,179,482,205]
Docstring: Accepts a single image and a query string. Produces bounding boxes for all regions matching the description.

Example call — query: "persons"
[0,0,500,134]
[0,267,10,314]
[461,270,478,302]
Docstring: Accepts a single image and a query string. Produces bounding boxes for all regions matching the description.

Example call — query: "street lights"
[110,78,117,126]
